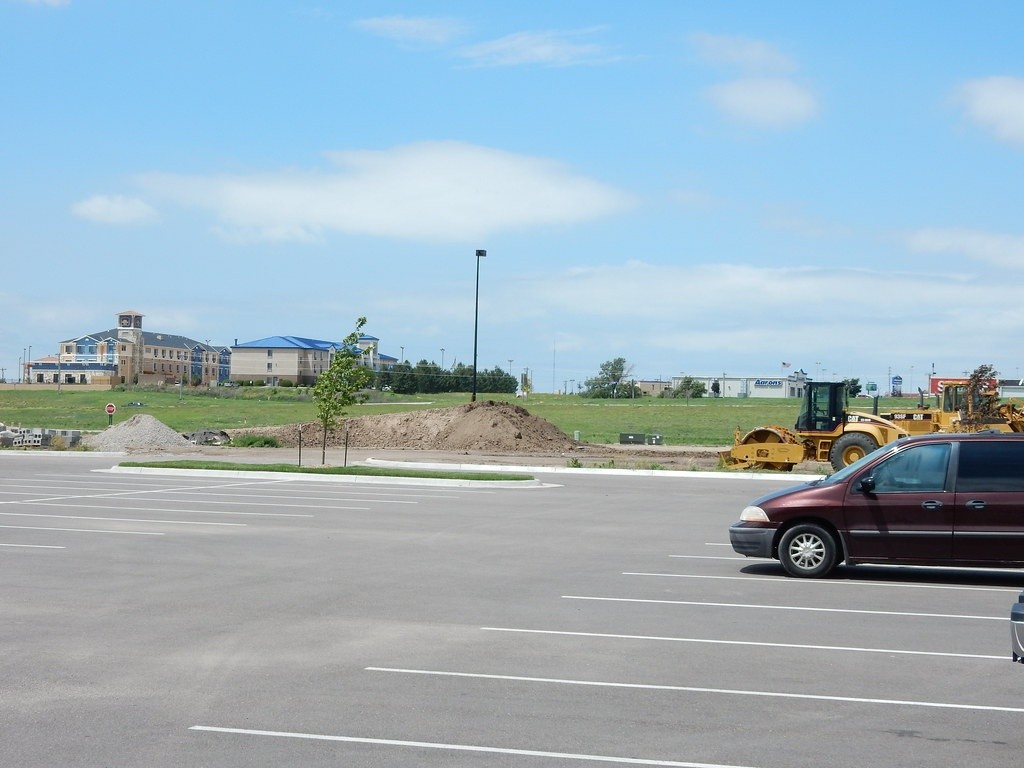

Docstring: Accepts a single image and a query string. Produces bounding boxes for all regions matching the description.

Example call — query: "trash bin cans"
[647,434,664,445]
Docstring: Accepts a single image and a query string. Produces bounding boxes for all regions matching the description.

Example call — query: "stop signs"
[105,403,116,414]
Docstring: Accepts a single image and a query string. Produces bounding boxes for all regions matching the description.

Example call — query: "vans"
[728,431,1024,578]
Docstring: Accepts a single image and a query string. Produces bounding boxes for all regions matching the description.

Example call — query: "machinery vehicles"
[718,382,911,474]
[877,380,1024,437]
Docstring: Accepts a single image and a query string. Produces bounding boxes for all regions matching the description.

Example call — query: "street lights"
[28,345,32,383]
[471,249,486,402]
[400,346,404,364]
[439,348,446,371]
[18,357,21,382]
[507,359,514,375]
[23,348,27,383]
